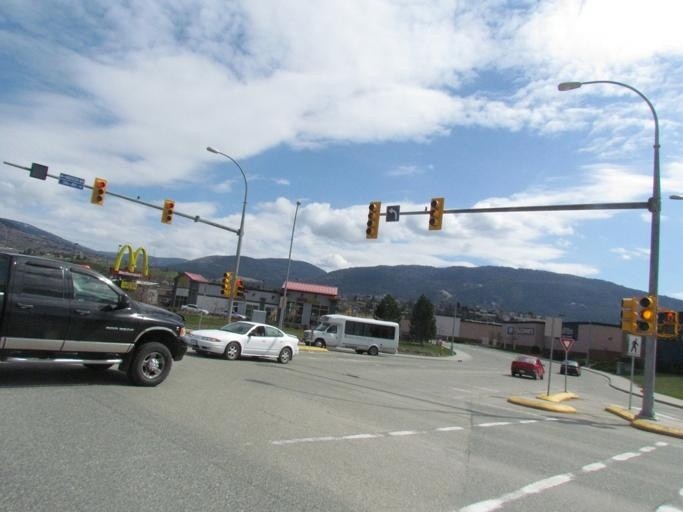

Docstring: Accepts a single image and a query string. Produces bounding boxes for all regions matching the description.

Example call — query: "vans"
[305,314,400,355]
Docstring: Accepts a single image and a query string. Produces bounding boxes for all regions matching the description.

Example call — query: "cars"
[182,304,210,315]
[561,360,581,375]
[511,356,544,379]
[185,322,299,363]
[223,310,247,320]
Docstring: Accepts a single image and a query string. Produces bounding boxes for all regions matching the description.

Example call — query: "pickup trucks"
[0,253,186,387]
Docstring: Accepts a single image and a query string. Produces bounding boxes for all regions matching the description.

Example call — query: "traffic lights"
[655,312,681,342]
[220,273,232,298]
[161,201,175,224]
[235,279,244,298]
[91,178,107,205]
[366,203,380,238]
[639,297,656,338]
[618,297,639,332]
[429,198,443,230]
